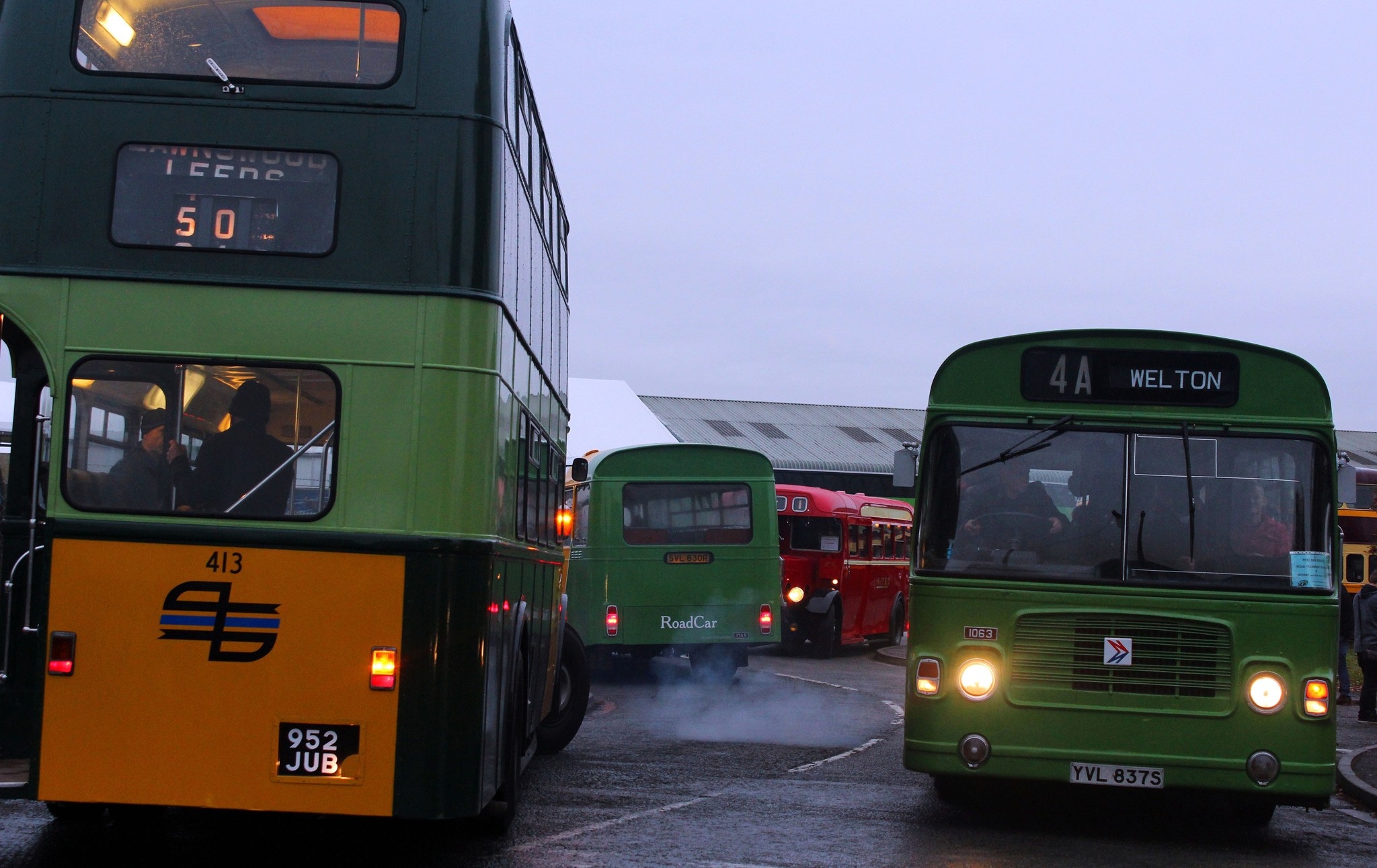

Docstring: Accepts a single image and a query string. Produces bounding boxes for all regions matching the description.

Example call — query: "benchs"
[624,527,672,544]
[691,530,706,545]
[669,530,694,544]
[705,528,752,545]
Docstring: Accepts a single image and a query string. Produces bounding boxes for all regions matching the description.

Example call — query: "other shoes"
[1358,710,1377,723]
[1336,694,1351,706]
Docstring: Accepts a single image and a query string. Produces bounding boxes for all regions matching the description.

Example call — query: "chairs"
[95,470,109,498]
[72,469,101,507]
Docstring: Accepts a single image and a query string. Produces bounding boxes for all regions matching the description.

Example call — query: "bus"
[709,484,916,660]
[0,0,588,851]
[560,440,785,678]
[892,325,1357,826]
[1333,467,1377,601]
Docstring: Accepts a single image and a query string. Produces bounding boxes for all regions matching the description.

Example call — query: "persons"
[102,407,193,514]
[1336,582,1353,706]
[1171,475,1292,565]
[167,380,295,516]
[1353,568,1377,725]
[960,454,1070,565]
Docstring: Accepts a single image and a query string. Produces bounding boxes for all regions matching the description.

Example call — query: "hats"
[228,379,271,424]
[141,408,166,435]
